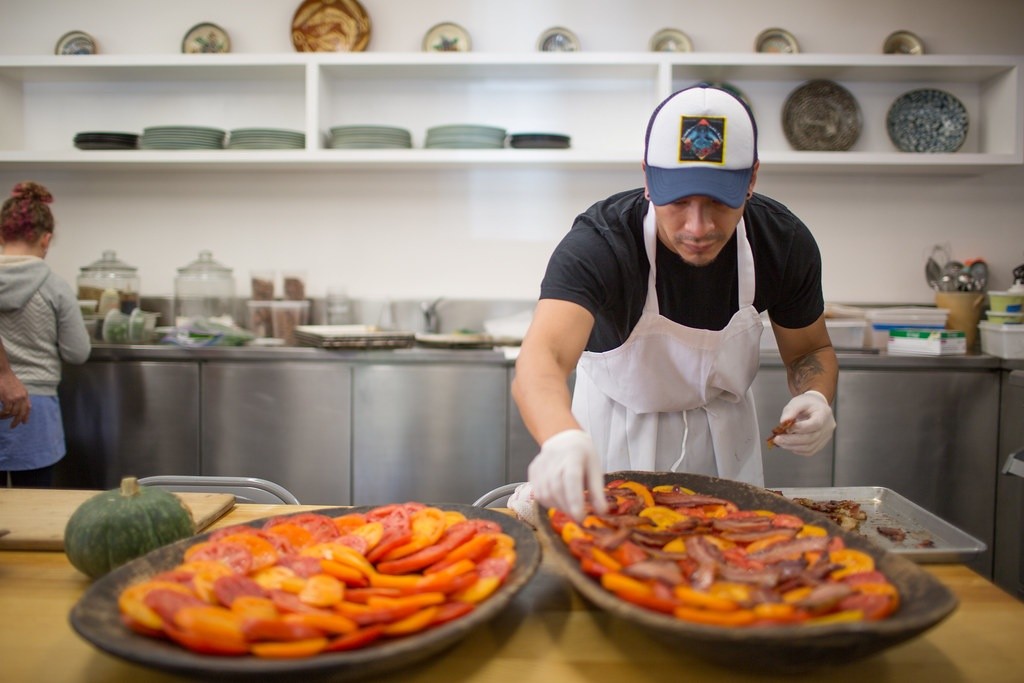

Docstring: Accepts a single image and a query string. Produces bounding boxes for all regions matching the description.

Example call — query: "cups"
[244,300,310,344]
[935,292,988,355]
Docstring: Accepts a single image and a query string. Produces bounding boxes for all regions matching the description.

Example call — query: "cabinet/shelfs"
[0,51,1023,175]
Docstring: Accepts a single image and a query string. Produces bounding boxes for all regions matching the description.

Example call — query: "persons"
[508,80,838,522]
[0,182,92,490]
[0,337,31,428]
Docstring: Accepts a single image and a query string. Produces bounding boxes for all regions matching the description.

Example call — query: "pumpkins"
[65,477,193,580]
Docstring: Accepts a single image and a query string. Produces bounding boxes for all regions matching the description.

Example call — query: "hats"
[642,82,758,209]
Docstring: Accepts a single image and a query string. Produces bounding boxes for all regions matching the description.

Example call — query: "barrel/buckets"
[863,307,949,350]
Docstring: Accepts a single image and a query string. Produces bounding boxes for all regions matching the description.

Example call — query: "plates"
[535,472,957,674]
[73,127,307,151]
[69,503,544,683]
[53,0,924,57]
[886,88,970,154]
[780,79,864,151]
[294,323,522,348]
[329,124,575,150]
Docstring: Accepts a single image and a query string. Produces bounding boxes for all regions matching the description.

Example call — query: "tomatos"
[116,498,517,658]
[546,480,900,629]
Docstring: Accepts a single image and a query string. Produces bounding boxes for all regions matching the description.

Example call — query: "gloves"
[771,391,838,456]
[527,430,607,518]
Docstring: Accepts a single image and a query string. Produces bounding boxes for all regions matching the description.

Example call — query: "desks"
[0,504,1024,683]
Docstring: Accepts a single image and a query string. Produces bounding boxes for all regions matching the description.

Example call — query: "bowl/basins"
[979,291,1024,359]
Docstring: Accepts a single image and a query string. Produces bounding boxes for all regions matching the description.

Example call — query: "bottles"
[75,250,139,312]
[173,252,237,320]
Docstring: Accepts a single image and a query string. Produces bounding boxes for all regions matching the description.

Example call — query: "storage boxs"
[977,320,1024,360]
[888,329,967,354]
